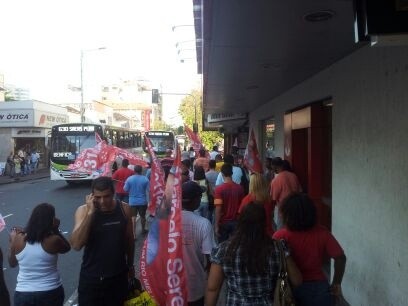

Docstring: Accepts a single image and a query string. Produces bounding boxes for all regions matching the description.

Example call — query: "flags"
[243,124,264,173]
[141,138,191,306]
[142,134,168,218]
[184,123,211,159]
[69,136,149,174]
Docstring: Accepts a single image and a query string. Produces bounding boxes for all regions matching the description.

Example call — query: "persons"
[203,201,302,306]
[237,172,276,239]
[72,176,136,306]
[10,203,72,306]
[123,164,152,242]
[141,179,225,306]
[273,192,348,306]
[0,125,282,221]
[212,163,245,244]
[269,156,305,230]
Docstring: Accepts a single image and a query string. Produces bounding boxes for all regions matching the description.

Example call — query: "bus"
[176,135,186,152]
[45,123,143,185]
[143,131,178,161]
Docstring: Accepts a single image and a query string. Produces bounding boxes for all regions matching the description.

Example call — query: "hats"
[181,181,208,203]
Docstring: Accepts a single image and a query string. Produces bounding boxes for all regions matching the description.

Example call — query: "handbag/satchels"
[123,278,158,306]
[274,241,294,306]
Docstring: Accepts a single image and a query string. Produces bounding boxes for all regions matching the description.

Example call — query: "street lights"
[81,47,106,122]
